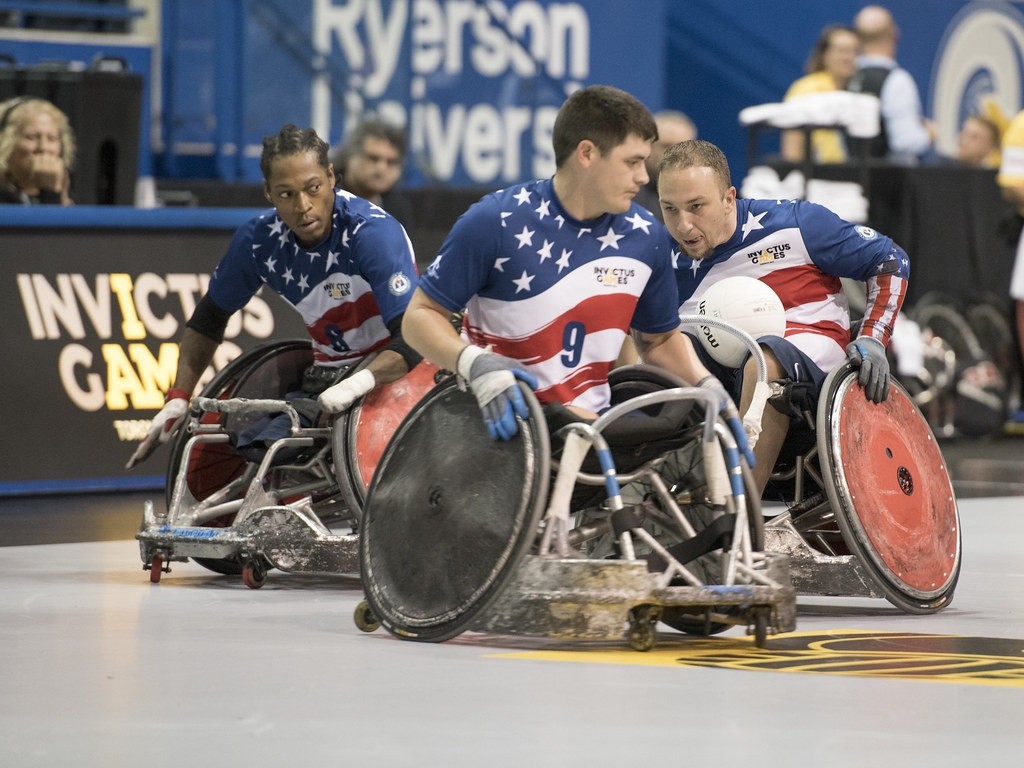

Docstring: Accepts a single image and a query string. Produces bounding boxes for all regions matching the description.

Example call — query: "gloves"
[696,376,755,468]
[317,367,375,414]
[846,336,890,405]
[456,344,538,440]
[125,389,189,468]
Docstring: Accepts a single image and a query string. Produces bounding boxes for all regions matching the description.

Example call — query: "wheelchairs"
[135,338,389,588]
[355,354,798,652]
[676,319,962,616]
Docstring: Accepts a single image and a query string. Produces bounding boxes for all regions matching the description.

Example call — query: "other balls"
[694,278,786,368]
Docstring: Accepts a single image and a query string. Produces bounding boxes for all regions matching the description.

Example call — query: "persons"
[0,96,79,210]
[625,141,911,561]
[122,108,421,518]
[401,84,754,514]
[776,4,999,171]
